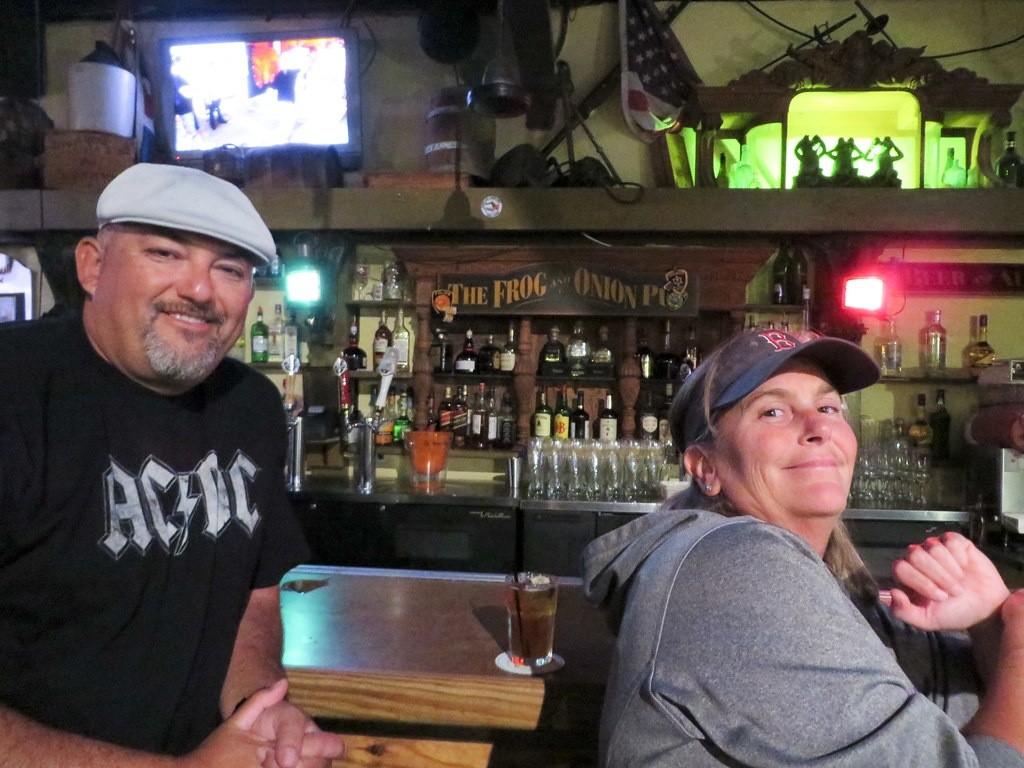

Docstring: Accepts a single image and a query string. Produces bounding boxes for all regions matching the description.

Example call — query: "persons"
[0,162,346,767]
[579,330,1024,767]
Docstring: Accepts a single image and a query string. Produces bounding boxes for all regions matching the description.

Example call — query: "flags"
[618,0,691,145]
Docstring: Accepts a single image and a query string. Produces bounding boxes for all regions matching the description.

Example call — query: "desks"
[278,562,634,768]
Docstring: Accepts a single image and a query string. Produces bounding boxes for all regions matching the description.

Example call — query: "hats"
[674,327,882,447]
[95,162,276,266]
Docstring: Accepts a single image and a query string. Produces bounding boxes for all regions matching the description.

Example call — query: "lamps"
[467,1,528,120]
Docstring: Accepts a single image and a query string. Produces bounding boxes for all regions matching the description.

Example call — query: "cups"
[839,391,881,446]
[527,437,668,499]
[372,262,404,301]
[509,458,524,488]
[405,432,453,495]
[505,573,557,667]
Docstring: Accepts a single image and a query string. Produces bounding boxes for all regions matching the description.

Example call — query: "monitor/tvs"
[158,28,364,172]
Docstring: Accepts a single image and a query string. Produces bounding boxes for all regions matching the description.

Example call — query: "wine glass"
[850,446,928,505]
[353,259,369,300]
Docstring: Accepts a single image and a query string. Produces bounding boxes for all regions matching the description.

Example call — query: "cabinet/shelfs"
[226,238,1023,505]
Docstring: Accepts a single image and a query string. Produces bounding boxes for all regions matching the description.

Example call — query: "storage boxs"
[42,131,139,193]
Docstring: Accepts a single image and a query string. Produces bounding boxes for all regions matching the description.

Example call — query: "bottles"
[941,148,966,188]
[342,309,410,372]
[970,494,986,546]
[887,389,951,468]
[252,244,281,276]
[431,320,702,380]
[347,382,518,451]
[968,315,995,376]
[925,311,946,375]
[640,383,673,441]
[717,153,729,188]
[881,321,902,377]
[970,132,1024,188]
[772,240,808,305]
[228,305,300,362]
[748,289,825,343]
[282,378,297,419]
[532,385,618,441]
